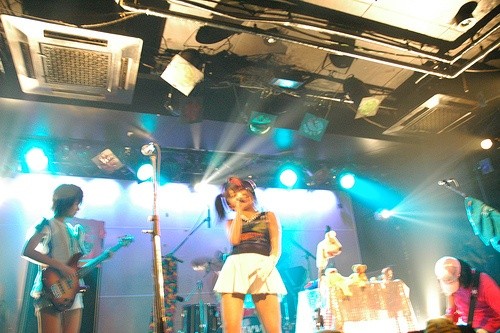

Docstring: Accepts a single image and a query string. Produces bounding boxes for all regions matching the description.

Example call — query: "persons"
[424,317,464,333]
[213,177,287,333]
[191,259,224,286]
[434,257,500,333]
[23,184,103,333]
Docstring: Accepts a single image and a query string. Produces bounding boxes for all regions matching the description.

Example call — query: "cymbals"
[190,256,223,271]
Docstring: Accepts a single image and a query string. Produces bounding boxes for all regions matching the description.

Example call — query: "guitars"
[41,234,135,312]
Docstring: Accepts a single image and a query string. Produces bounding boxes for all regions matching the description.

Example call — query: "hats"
[434,256,461,296]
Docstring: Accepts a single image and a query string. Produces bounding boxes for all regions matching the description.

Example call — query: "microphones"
[438,178,454,185]
[141,143,154,155]
[207,208,211,228]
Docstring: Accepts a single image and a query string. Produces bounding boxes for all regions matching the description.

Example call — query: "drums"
[180,303,221,333]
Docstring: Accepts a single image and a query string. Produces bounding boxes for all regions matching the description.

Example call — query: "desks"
[295,279,421,333]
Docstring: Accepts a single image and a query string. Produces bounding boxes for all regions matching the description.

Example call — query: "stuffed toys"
[317,232,394,285]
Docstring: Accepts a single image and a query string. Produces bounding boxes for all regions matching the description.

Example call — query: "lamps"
[343,76,387,120]
[271,71,306,90]
[373,207,391,224]
[480,137,498,155]
[242,101,280,138]
[125,156,154,182]
[296,105,331,143]
[159,48,206,97]
